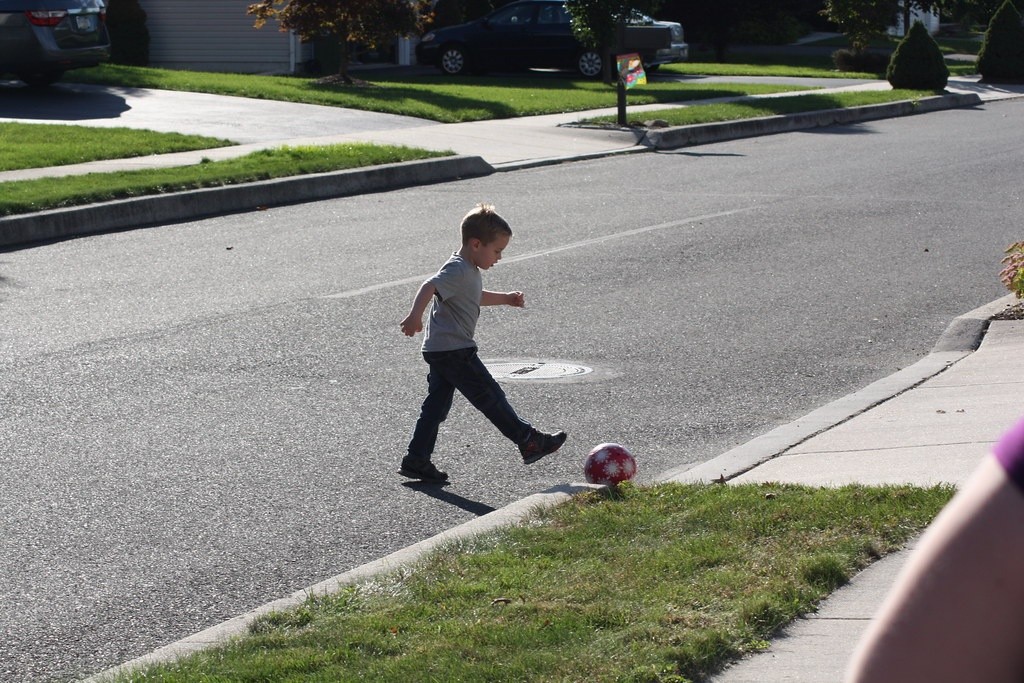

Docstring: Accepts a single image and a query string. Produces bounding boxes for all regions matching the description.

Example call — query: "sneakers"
[518,428,567,465]
[397,456,448,482]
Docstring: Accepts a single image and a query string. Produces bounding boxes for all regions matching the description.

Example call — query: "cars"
[415,0,690,77]
[0,0,112,85]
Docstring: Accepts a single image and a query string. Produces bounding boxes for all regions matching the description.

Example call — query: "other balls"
[584,443,636,486]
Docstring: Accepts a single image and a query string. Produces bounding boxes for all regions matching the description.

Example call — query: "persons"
[395,202,567,481]
[842,416,1023,682]
